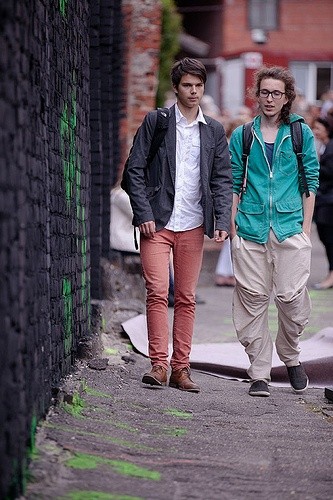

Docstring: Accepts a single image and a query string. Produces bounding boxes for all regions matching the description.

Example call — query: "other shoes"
[313,279,333,291]
[248,380,271,397]
[141,366,167,387]
[287,362,309,394]
[167,368,200,392]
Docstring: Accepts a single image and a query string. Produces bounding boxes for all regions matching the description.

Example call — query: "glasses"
[258,89,286,101]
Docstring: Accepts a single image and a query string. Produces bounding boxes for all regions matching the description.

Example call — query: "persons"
[229,64,319,396]
[167,89,333,307]
[126,57,234,391]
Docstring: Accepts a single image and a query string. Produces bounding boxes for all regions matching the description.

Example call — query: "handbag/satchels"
[120,108,169,250]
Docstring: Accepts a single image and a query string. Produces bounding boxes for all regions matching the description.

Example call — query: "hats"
[317,113,333,135]
